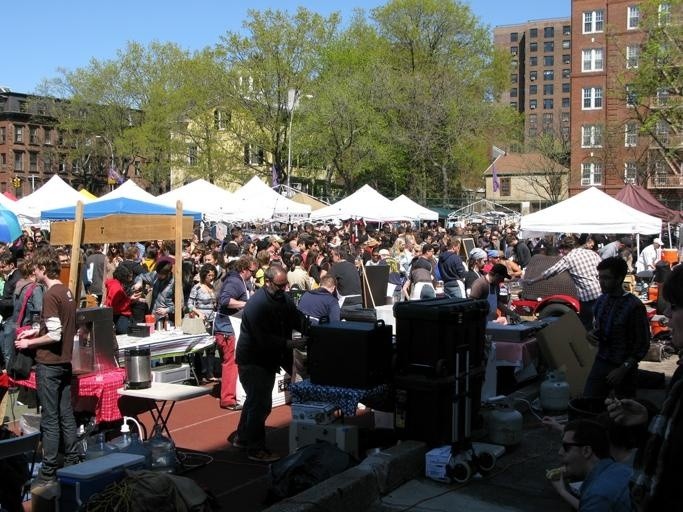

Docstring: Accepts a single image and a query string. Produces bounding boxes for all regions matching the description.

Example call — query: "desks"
[1,364,124,424]
[115,326,216,385]
[117,381,210,448]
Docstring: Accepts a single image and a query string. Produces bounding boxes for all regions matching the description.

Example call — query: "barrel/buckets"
[109,430,134,449]
[119,433,152,467]
[85,433,119,461]
[144,424,176,468]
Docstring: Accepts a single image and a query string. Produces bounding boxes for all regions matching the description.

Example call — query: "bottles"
[188,308,195,318]
[164,314,171,331]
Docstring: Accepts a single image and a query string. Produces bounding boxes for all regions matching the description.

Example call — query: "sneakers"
[233,434,279,461]
[226,404,242,410]
[22,479,61,494]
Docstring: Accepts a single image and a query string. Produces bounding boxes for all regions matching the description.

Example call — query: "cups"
[145,314,155,334]
[156,321,163,331]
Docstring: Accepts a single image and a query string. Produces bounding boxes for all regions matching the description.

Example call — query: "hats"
[653,238,664,246]
[493,264,511,279]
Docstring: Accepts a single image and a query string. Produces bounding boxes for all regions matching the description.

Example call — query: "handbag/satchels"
[16,326,36,350]
[7,348,33,379]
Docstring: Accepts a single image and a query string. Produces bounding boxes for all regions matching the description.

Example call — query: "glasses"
[596,275,615,282]
[271,281,287,289]
[562,443,577,453]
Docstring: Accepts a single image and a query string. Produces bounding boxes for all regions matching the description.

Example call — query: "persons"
[0,208,683,511]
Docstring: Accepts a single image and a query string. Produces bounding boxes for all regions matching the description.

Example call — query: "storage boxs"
[55,452,145,511]
